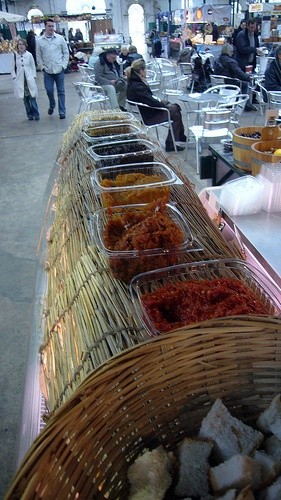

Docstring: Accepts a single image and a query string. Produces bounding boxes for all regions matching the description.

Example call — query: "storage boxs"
[251,139,281,176]
[232,124,281,170]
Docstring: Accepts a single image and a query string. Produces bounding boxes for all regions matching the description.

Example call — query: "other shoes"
[29,118,39,120]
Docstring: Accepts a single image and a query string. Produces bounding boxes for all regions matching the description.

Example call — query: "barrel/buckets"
[251,140,281,177]
[232,126,263,171]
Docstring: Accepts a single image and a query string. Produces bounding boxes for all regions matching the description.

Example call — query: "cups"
[246,66,251,74]
[248,65,253,73]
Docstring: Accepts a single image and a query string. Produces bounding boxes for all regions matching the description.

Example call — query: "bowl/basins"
[90,203,193,282]
[90,162,184,207]
[86,139,162,168]
[85,112,137,126]
[128,259,281,338]
[81,124,146,146]
[257,162,280,212]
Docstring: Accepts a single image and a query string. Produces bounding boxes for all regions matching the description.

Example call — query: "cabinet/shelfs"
[94,33,123,49]
[0,52,15,75]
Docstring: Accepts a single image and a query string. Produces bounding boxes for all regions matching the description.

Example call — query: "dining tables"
[177,92,222,156]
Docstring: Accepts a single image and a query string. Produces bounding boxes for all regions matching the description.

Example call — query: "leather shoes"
[166,145,185,152]
[48,108,53,114]
[119,106,127,111]
[60,114,65,119]
[179,136,190,142]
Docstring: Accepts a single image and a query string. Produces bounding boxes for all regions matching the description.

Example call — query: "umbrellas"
[0,10,26,26]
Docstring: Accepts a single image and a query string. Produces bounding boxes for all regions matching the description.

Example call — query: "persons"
[59,27,84,41]
[35,19,70,121]
[118,44,130,68]
[232,20,247,60]
[214,44,257,112]
[261,45,281,124]
[93,47,129,113]
[178,37,203,75]
[235,17,261,76]
[10,38,40,121]
[124,58,190,152]
[123,46,143,68]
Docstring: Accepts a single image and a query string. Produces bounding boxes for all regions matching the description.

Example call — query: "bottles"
[261,116,279,142]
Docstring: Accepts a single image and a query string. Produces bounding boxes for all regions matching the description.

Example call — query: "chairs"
[125,99,178,153]
[72,55,281,126]
[185,109,234,174]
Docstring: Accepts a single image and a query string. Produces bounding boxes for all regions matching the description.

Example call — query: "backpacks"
[214,59,239,90]
